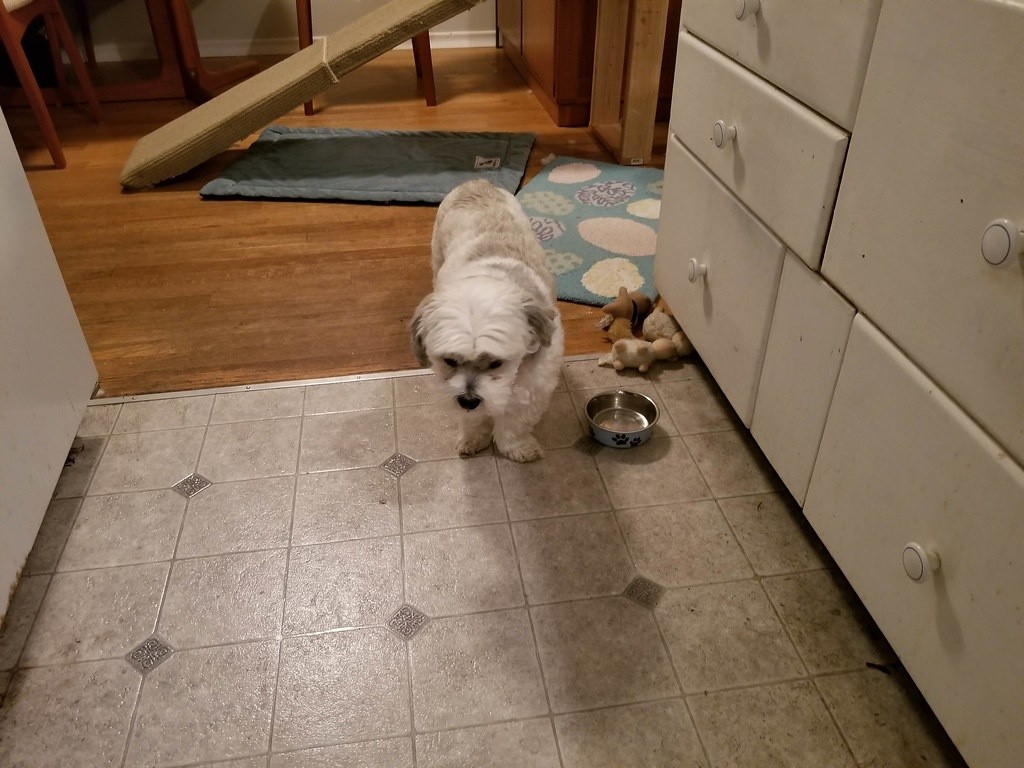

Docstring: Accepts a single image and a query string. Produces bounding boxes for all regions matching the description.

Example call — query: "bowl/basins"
[584,390,661,448]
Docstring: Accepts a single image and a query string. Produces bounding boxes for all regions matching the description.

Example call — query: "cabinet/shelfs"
[497,0,682,127]
[650,0,1024,768]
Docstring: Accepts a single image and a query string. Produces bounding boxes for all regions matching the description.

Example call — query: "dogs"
[408,178,565,461]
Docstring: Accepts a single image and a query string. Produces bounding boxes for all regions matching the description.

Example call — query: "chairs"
[0,0,103,169]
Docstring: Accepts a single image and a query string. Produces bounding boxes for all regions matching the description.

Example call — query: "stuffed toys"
[595,286,694,374]
